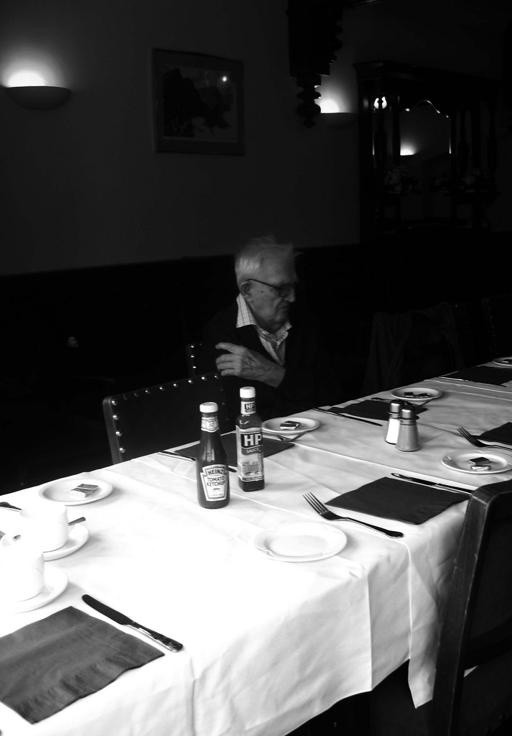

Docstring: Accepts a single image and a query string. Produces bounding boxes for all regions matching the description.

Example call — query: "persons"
[203,241,333,429]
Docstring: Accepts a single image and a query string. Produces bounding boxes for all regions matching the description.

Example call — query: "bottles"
[384,399,402,445]
[196,402,230,509]
[396,406,421,452]
[235,386,265,491]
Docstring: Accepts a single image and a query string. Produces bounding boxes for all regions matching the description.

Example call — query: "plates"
[388,385,442,404]
[0,564,75,615]
[261,415,320,437]
[3,525,89,561]
[35,477,115,507]
[443,446,511,479]
[256,520,349,563]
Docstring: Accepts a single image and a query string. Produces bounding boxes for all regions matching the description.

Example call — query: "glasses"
[245,278,293,297]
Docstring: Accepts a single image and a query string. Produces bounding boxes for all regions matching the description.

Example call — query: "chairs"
[101,372,234,464]
[387,477,509,729]
[184,339,233,380]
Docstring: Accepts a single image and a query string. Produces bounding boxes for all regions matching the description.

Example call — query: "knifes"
[156,448,237,478]
[80,592,184,659]
[312,406,382,433]
[391,470,474,499]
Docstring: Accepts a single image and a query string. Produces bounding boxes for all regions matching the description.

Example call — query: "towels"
[442,365,512,385]
[0,606,164,725]
[323,477,472,526]
[174,432,295,468]
[326,399,428,422]
[478,422,512,446]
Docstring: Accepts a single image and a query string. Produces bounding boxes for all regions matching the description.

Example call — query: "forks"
[303,491,405,548]
[456,424,511,451]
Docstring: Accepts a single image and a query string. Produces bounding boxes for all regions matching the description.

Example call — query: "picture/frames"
[150,47,247,158]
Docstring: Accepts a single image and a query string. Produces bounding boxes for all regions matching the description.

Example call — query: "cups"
[16,509,70,552]
[1,556,53,601]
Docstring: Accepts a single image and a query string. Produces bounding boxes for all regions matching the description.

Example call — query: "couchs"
[0,230,367,496]
